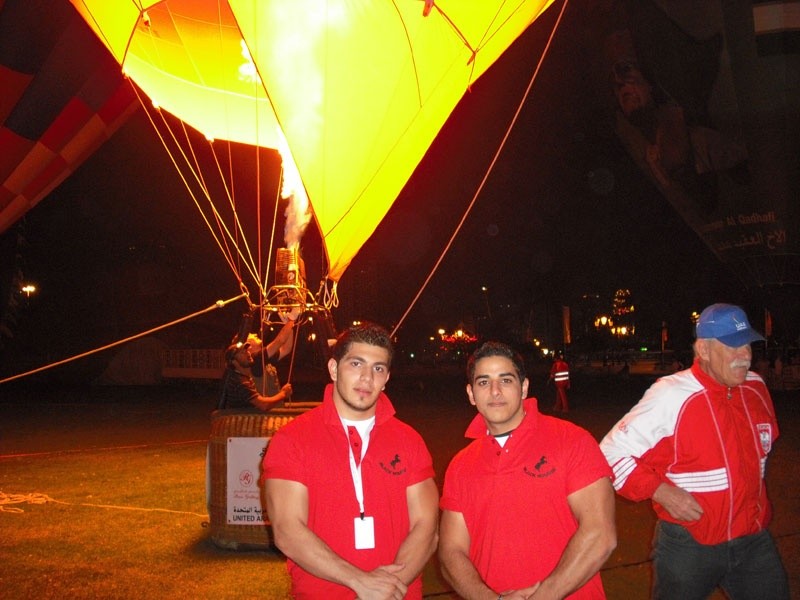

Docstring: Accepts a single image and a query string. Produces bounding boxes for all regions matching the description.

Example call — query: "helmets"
[555,349,563,358]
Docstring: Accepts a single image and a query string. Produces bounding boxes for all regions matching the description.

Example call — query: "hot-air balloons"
[63,0,560,552]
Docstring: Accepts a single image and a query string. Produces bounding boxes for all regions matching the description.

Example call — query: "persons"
[203,311,295,517]
[599,303,792,600]
[256,320,441,600]
[545,353,573,414]
[434,341,616,600]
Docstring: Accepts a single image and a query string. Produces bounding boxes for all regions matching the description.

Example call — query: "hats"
[224,342,251,363]
[695,302,767,348]
